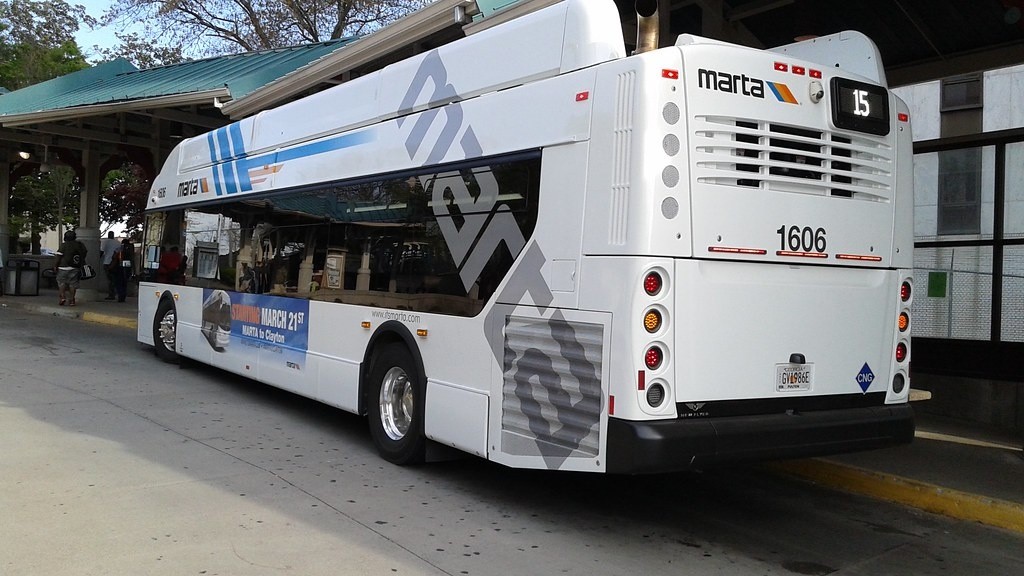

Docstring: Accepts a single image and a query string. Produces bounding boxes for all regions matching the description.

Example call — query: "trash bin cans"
[4,256,41,297]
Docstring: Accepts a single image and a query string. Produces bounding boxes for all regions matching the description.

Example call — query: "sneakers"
[59,295,67,306]
[69,299,76,307]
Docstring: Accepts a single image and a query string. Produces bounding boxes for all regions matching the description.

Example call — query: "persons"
[100,230,135,303]
[144,245,188,275]
[53,230,87,306]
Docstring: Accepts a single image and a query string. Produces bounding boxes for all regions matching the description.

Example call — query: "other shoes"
[117,294,126,303]
[104,293,116,301]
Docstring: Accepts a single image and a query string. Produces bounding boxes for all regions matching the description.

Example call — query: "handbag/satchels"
[123,260,130,267]
[107,251,120,271]
[78,264,97,281]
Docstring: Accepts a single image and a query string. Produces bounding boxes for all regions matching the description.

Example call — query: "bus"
[136,0,933,474]
[202,290,231,348]
